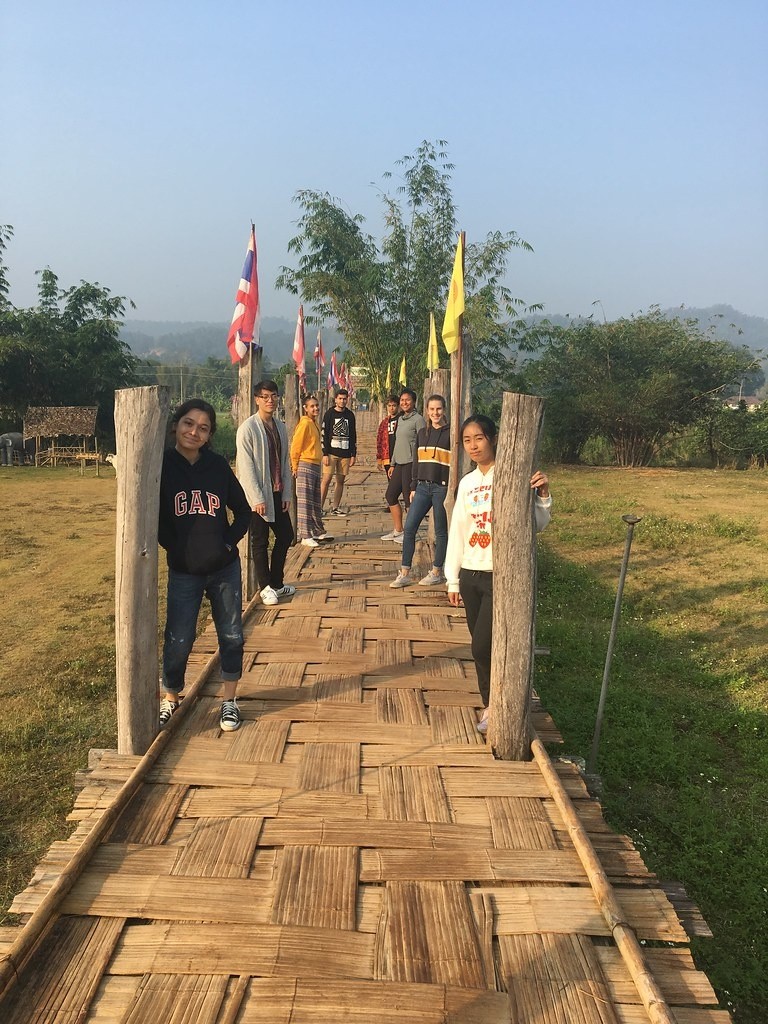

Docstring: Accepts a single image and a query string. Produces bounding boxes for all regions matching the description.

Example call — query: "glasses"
[259,394,280,403]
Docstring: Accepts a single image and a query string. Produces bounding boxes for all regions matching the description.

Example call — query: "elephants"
[0,432,36,466]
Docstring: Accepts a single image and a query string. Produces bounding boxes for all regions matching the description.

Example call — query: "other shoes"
[313,533,334,541]
[382,507,391,514]
[477,717,488,734]
[301,537,319,547]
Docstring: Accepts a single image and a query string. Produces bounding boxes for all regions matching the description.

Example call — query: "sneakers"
[159,699,180,726]
[275,584,295,598]
[394,533,418,543]
[220,697,241,732]
[419,570,444,586]
[389,570,410,588]
[321,510,327,516]
[260,585,279,605]
[381,529,405,540]
[330,507,347,517]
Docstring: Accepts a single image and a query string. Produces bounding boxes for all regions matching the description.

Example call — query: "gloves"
[410,480,417,491]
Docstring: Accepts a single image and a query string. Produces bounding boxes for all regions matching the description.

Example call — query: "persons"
[235,380,295,606]
[444,414,552,736]
[377,394,399,512]
[389,394,450,588]
[321,389,356,517]
[366,401,369,411]
[160,398,252,731]
[381,389,427,544]
[288,393,334,545]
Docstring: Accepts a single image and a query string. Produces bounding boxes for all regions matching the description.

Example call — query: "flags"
[441,237,464,354]
[328,353,354,397]
[427,317,439,372]
[377,367,391,393]
[227,234,259,364]
[314,335,326,374]
[400,358,406,387]
[293,308,305,387]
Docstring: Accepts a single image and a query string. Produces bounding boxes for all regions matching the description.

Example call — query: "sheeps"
[105,453,118,480]
